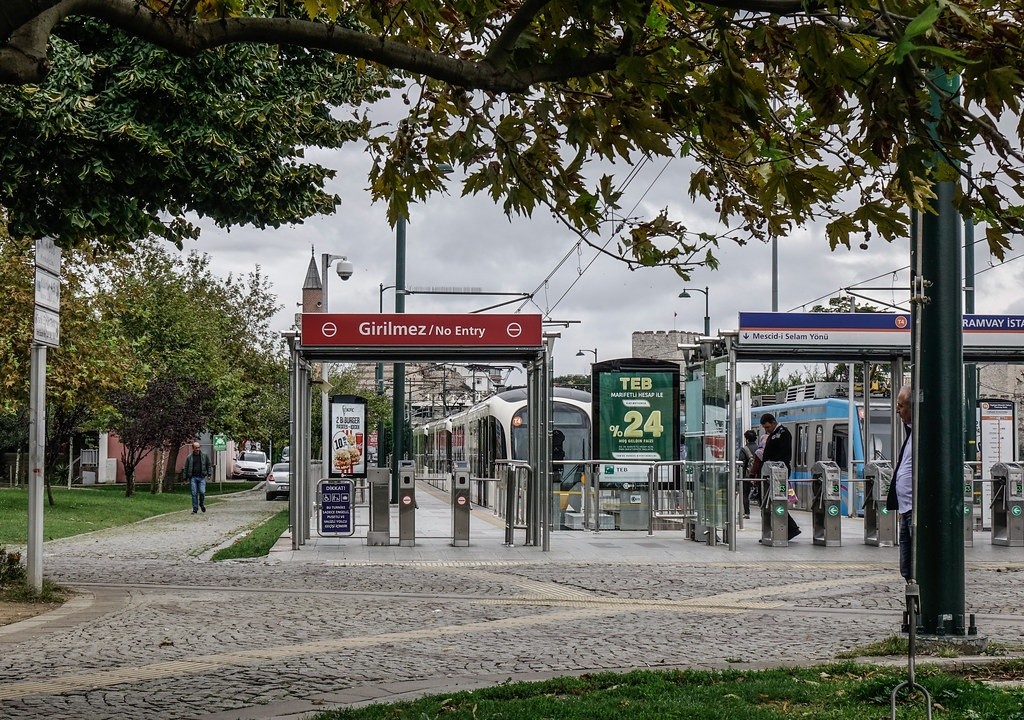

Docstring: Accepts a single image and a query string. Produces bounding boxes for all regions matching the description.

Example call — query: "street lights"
[576,348,597,364]
[678,286,711,337]
[320,252,354,482]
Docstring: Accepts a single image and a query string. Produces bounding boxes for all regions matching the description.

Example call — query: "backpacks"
[741,446,757,479]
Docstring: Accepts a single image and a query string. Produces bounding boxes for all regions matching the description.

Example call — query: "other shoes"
[738,511,750,519]
[788,528,801,541]
[199,504,206,512]
[191,511,197,515]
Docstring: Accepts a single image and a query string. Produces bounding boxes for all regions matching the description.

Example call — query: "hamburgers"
[333,448,361,470]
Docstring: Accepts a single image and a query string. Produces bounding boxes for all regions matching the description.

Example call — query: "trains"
[409,384,593,515]
[736,379,896,518]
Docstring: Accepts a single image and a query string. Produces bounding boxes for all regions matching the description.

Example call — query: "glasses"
[896,401,904,408]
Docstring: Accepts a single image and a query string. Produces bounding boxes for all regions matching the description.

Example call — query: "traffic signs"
[32,302,60,347]
[35,237,64,276]
[34,265,62,314]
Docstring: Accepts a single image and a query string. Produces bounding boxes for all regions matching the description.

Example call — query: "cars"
[266,444,290,499]
[232,449,271,481]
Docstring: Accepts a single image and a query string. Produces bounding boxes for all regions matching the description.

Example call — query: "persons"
[886,384,914,585]
[184,442,213,515]
[740,413,802,541]
